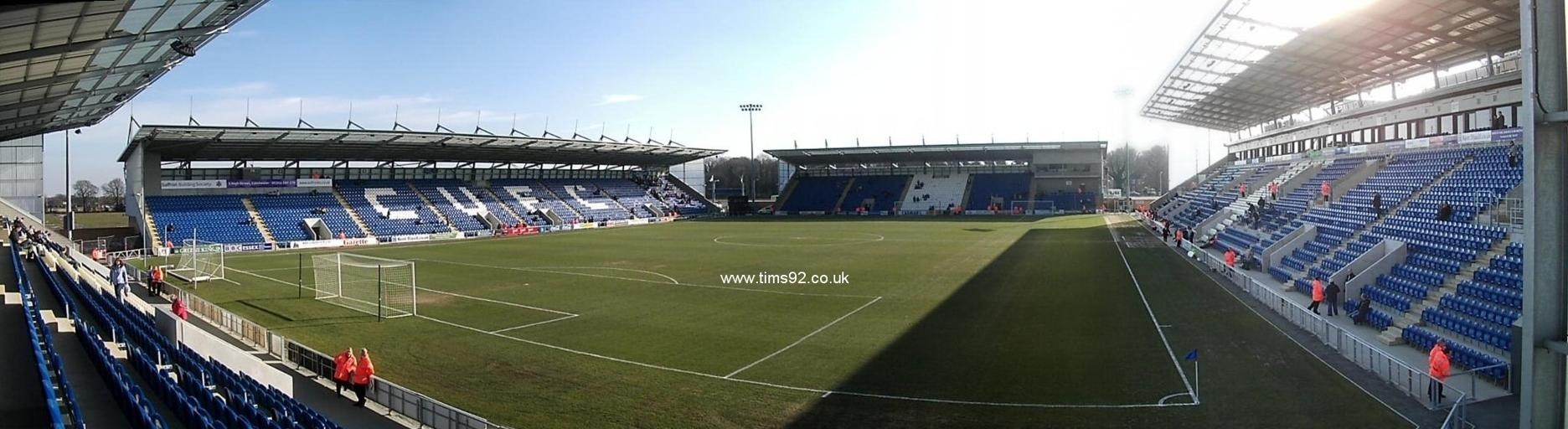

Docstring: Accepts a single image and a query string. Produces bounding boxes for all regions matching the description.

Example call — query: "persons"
[1,212,189,321]
[1370,191,1381,219]
[631,170,698,214]
[1428,335,1446,397]
[1434,201,1451,220]
[1496,111,1504,130]
[354,347,378,407]
[753,181,1146,217]
[1344,267,1355,285]
[1145,171,1332,271]
[1506,140,1516,167]
[1426,347,1454,404]
[1306,276,1324,315]
[1492,114,1499,126]
[333,346,358,395]
[1349,292,1370,324]
[1324,279,1341,316]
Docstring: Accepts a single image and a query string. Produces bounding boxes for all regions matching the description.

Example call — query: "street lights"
[61,127,88,248]
[735,102,764,202]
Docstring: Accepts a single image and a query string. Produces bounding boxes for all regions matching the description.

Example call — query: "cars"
[1124,183,1160,198]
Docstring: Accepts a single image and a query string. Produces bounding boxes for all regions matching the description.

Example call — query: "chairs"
[146,192,366,244]
